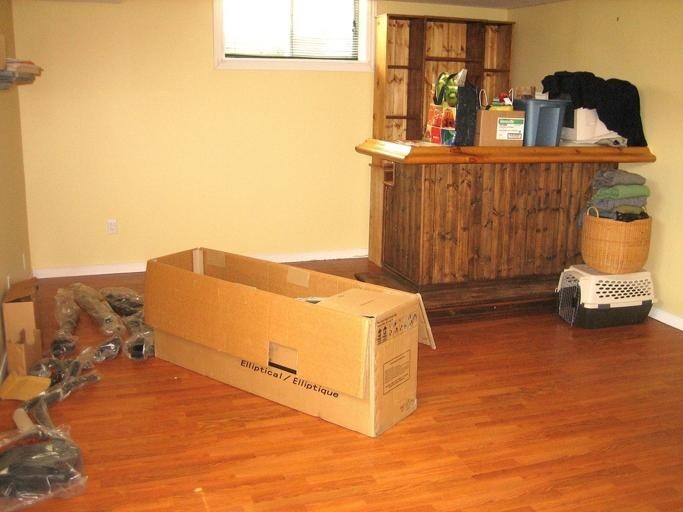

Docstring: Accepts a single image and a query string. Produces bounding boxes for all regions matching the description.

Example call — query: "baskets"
[582,207,652,275]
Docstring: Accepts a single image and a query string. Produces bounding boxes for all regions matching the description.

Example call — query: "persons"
[432,109,441,128]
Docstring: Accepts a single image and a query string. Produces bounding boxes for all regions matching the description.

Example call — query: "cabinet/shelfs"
[373,12,516,147]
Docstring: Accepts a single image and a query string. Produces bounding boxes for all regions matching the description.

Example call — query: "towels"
[591,168,649,208]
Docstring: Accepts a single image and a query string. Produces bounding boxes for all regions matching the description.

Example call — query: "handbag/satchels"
[479,88,514,111]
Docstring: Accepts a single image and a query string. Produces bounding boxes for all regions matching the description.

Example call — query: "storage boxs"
[4,278,40,347]
[472,98,573,148]
[145,246,440,441]
